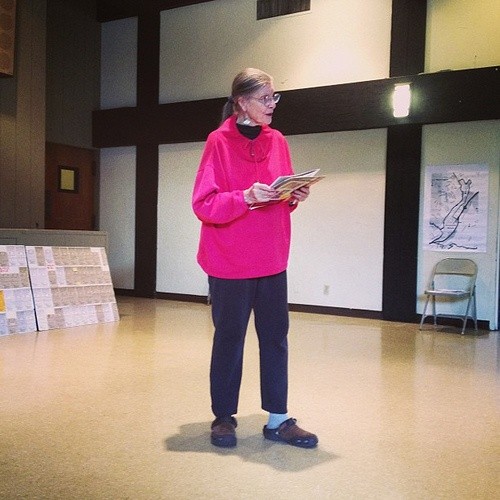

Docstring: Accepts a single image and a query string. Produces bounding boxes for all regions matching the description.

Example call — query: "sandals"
[211,417,238,446]
[263,418,318,448]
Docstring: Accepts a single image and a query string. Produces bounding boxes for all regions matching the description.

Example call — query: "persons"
[192,68,319,449]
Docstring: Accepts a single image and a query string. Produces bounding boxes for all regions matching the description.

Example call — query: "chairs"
[419,258,478,335]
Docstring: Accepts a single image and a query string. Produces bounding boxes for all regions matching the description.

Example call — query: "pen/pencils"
[289,198,303,209]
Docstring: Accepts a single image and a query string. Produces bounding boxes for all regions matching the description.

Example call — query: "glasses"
[250,94,281,106]
